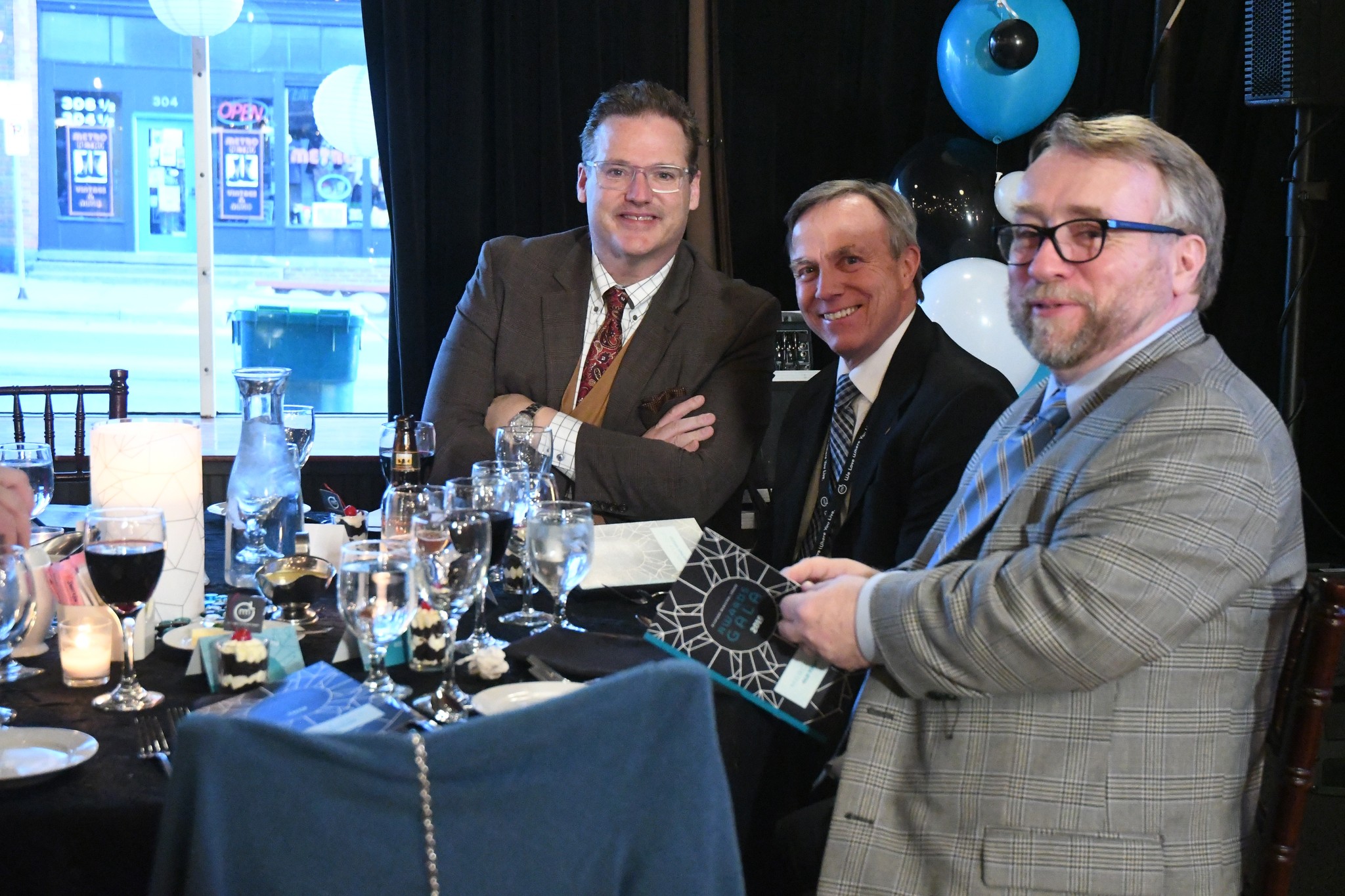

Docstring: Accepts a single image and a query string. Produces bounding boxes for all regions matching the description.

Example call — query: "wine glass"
[285,442,301,484]
[380,420,437,480]
[82,506,166,713]
[30,526,65,546]
[233,491,284,563]
[472,461,530,508]
[338,538,419,699]
[0,544,36,726]
[414,508,492,716]
[528,501,595,634]
[447,475,515,655]
[283,404,315,469]
[382,485,450,565]
[497,470,558,627]
[0,654,46,683]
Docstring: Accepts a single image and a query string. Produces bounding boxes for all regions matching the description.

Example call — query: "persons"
[711,177,1020,837]
[391,82,782,638]
[773,106,1308,896]
[0,464,36,557]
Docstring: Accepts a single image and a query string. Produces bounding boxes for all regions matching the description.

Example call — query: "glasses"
[585,159,690,194]
[996,217,1185,267]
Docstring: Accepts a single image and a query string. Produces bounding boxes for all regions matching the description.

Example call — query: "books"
[644,527,840,735]
[182,660,417,736]
[568,515,707,592]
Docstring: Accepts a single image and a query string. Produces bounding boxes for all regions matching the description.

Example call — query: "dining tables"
[0,515,764,896]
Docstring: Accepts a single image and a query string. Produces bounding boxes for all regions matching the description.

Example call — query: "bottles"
[391,414,423,487]
[222,367,305,589]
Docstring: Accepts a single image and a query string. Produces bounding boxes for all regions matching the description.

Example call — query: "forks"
[131,716,173,781]
[524,654,569,682]
[166,705,192,742]
[602,583,670,605]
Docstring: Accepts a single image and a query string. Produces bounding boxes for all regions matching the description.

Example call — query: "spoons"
[296,627,336,636]
[635,615,652,629]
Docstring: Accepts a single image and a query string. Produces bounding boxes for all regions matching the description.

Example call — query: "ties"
[925,390,1070,571]
[575,285,630,408]
[794,372,861,562]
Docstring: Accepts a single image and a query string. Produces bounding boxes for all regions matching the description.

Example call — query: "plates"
[163,620,305,650]
[1,726,102,786]
[471,682,586,717]
[206,501,312,516]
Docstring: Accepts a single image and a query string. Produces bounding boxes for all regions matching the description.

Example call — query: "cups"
[497,425,553,593]
[9,546,52,656]
[331,510,369,542]
[216,636,270,694]
[256,555,337,625]
[58,617,113,688]
[89,417,203,624]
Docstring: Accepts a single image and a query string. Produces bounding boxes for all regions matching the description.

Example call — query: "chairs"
[0,370,131,504]
[155,658,745,896]
[1240,573,1344,896]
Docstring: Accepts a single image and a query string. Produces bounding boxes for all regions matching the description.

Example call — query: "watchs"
[508,401,545,452]
